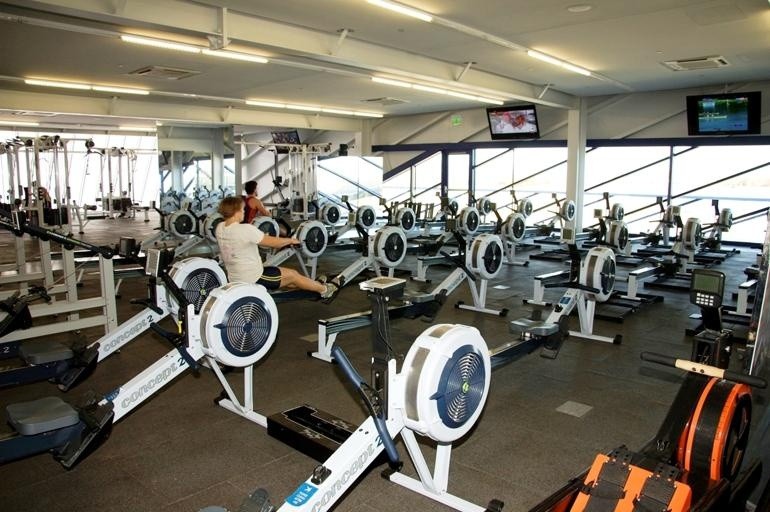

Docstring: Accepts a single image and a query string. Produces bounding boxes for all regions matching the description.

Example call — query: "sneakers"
[316,275,341,299]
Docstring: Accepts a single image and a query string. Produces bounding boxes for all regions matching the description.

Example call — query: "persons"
[502,110,539,132]
[31,180,52,209]
[241,180,297,239]
[495,112,513,133]
[275,132,298,151]
[14,198,22,211]
[213,195,339,300]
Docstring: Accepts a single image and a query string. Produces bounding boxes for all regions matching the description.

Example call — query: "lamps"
[528,50,591,77]
[91,86,149,95]
[371,77,505,106]
[120,35,201,53]
[200,49,268,63]
[370,0,432,23]
[245,101,384,118]
[26,79,91,90]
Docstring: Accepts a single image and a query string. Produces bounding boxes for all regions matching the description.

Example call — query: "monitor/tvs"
[485,104,540,139]
[271,131,302,154]
[685,90,762,136]
